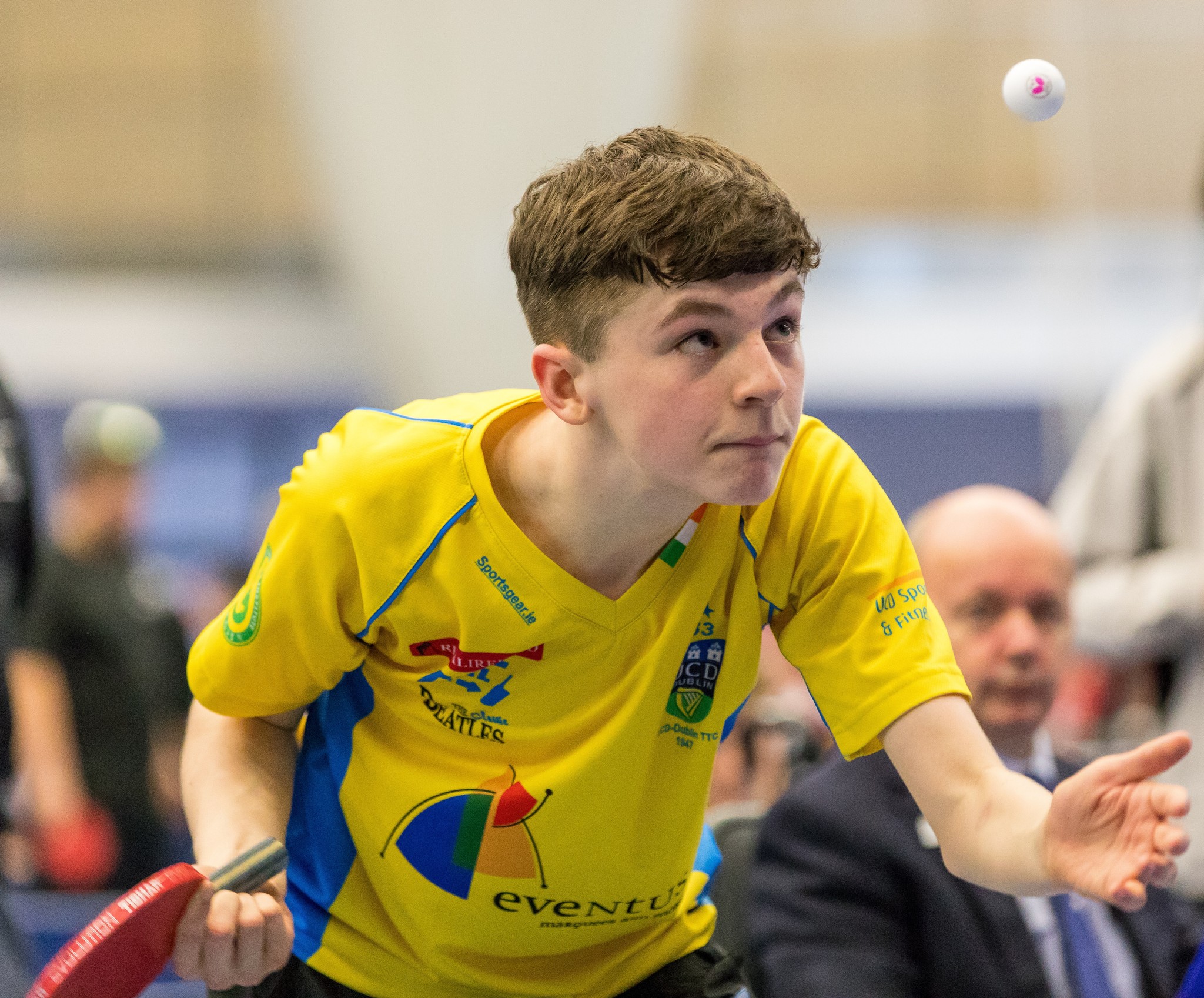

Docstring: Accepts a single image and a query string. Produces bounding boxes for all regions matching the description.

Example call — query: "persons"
[0,389,35,893]
[1049,171,1203,998]
[179,128,1190,998]
[9,436,185,895]
[743,484,1203,998]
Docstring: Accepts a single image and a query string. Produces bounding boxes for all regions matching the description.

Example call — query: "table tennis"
[1003,59,1067,121]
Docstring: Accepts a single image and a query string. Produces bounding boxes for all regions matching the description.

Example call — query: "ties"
[1026,773,1113,998]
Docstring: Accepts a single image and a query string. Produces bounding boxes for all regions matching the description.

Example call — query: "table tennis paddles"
[22,834,290,998]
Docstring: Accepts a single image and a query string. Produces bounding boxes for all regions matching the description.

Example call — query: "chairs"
[696,809,778,980]
